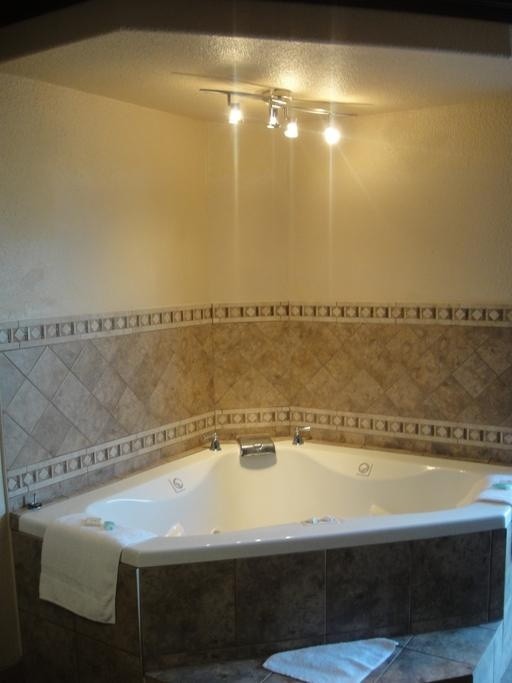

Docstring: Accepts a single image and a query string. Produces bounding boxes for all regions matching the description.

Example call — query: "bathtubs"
[19,439,512,668]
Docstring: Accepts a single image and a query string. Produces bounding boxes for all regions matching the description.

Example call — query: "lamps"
[227,88,356,145]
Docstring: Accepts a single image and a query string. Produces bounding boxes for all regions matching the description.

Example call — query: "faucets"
[210,431,220,451]
[292,424,311,445]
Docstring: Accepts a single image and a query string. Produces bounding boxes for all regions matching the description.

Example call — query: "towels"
[261,636,399,683]
[38,512,159,625]
[457,473,512,508]
